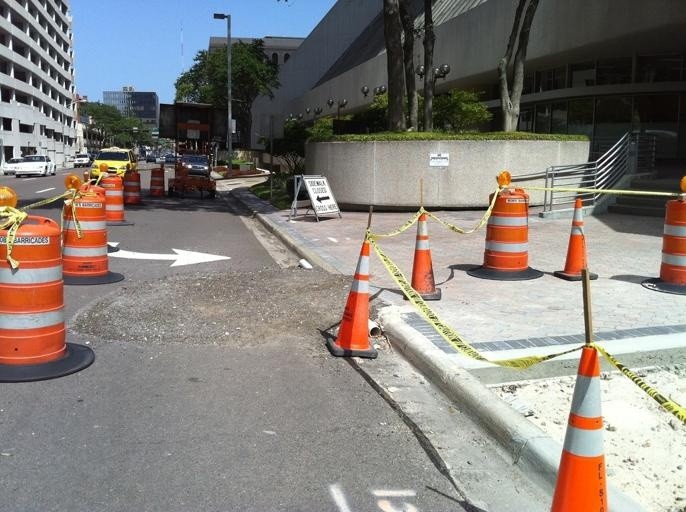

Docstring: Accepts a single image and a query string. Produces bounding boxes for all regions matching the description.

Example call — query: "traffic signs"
[305,177,340,214]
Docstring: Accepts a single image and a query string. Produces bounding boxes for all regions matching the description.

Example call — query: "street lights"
[62,98,88,165]
[212,13,233,179]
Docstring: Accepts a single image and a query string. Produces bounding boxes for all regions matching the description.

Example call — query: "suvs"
[74,153,91,167]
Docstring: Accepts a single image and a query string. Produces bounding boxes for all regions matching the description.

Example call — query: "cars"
[179,153,213,179]
[15,155,56,178]
[90,148,139,179]
[140,144,180,165]
[3,157,22,175]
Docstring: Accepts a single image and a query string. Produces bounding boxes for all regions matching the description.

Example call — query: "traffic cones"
[326,237,379,357]
[404,209,443,301]
[551,343,611,511]
[552,197,599,281]
